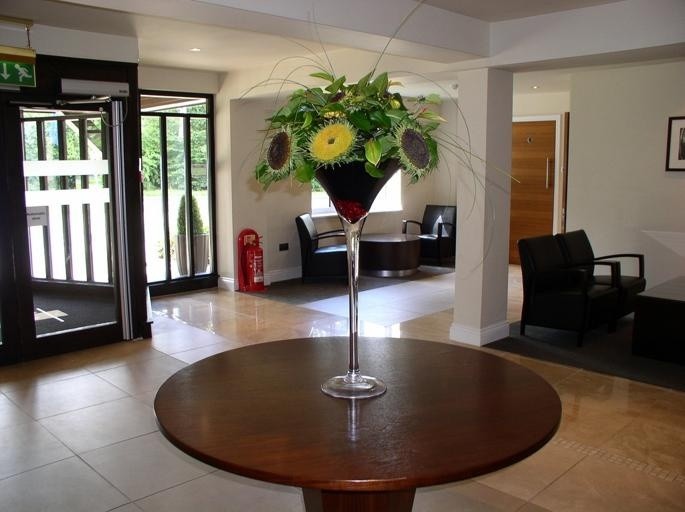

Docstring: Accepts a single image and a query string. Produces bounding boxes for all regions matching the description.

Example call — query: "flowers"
[235,0,523,276]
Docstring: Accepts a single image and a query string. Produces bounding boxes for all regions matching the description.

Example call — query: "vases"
[309,160,401,401]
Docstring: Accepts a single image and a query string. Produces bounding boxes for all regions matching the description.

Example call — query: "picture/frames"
[662,115,683,173]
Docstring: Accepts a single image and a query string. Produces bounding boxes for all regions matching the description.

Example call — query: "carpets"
[481,318,685,395]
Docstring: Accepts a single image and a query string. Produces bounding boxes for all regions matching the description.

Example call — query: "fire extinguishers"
[241,236,264,287]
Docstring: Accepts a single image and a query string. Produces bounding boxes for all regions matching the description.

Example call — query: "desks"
[154,334,562,512]
[631,275,683,356]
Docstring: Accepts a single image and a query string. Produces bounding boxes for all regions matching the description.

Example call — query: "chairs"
[513,227,648,352]
[294,201,457,289]
